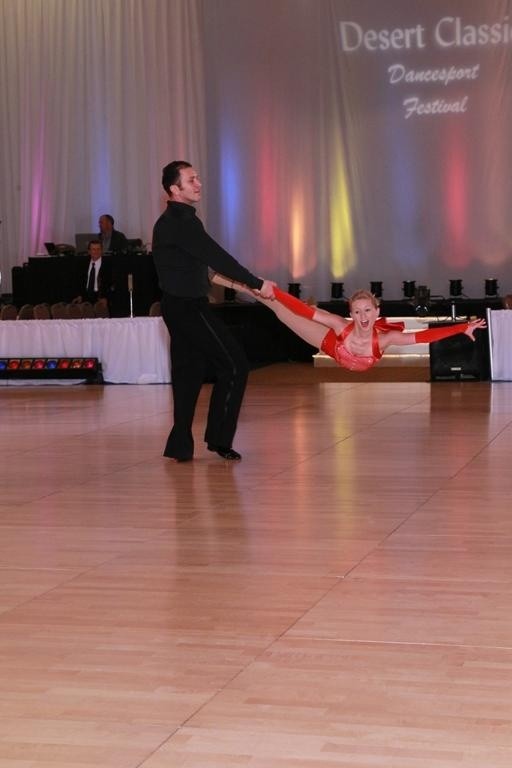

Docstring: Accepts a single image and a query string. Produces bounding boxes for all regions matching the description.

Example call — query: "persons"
[77,240,117,307]
[95,214,129,252]
[152,161,278,464]
[207,265,489,373]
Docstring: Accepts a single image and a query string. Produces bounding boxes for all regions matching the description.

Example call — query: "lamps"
[331,282,345,299]
[402,281,416,298]
[369,282,383,297]
[484,279,498,297]
[287,282,301,299]
[449,279,463,296]
[0,358,104,385]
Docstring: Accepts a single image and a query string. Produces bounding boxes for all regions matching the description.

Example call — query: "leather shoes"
[175,455,193,464]
[207,442,242,461]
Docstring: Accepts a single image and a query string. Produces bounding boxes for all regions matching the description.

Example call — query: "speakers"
[427,322,491,383]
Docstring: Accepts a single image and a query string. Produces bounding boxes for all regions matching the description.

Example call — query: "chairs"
[0,302,110,320]
[149,301,162,316]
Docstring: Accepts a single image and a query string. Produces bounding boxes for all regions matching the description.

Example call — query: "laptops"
[44,242,60,254]
[75,233,100,253]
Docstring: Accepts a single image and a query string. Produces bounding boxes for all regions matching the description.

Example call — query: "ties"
[86,262,96,296]
